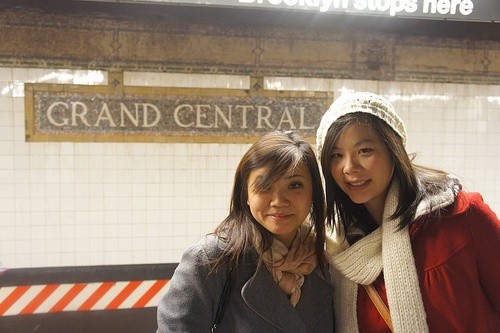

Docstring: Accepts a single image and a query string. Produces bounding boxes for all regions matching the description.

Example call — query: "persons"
[155,131,334,333]
[315,90,500,333]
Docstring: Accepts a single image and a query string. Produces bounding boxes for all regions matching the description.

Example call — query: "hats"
[313,91,407,164]
